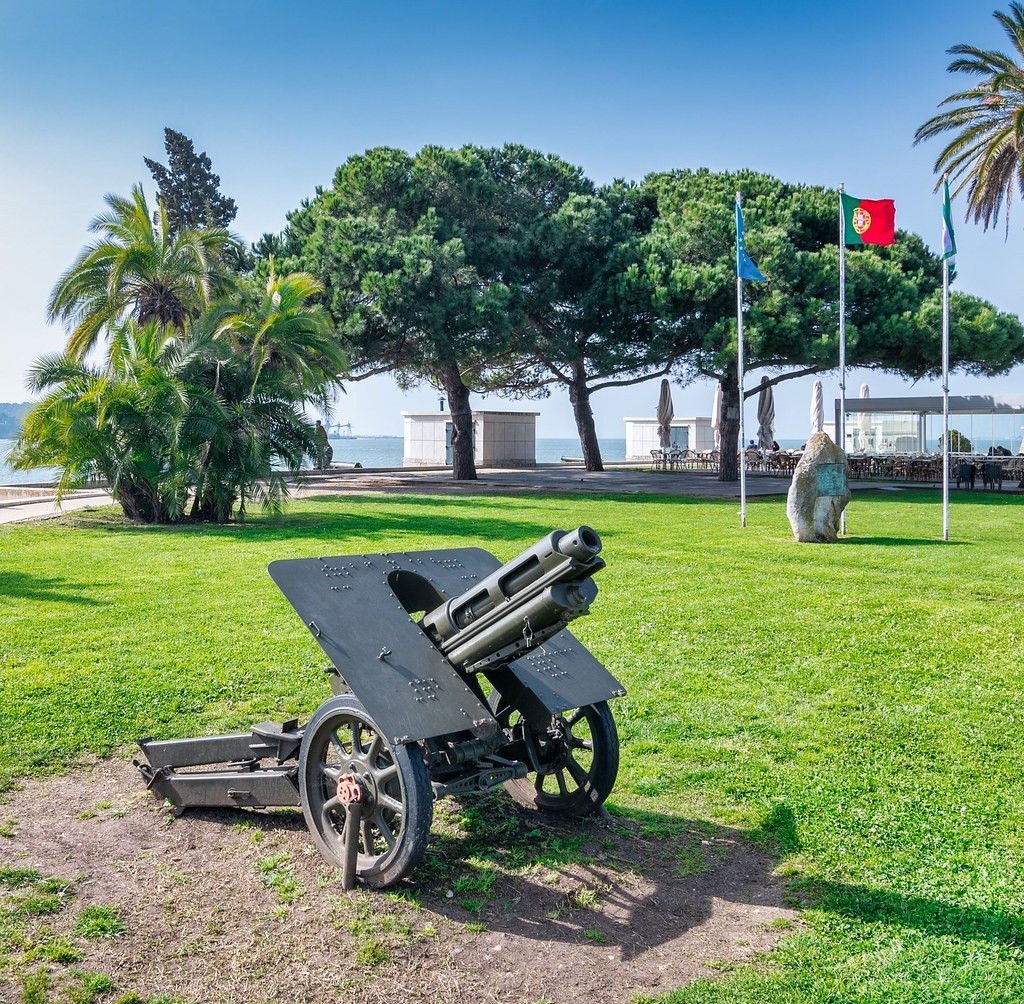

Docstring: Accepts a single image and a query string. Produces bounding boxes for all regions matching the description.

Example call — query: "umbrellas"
[757,375,776,467]
[656,379,674,469]
[711,382,722,472]
[858,384,872,454]
[811,380,824,434]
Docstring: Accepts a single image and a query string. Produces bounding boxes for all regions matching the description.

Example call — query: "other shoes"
[313,467,321,470]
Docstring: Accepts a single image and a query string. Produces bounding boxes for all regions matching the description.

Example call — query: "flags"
[735,201,767,282]
[942,181,957,270]
[841,193,896,246]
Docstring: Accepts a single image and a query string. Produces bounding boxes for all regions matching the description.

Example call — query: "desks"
[695,452,709,469]
[665,453,680,470]
[848,457,866,477]
[975,459,1008,483]
[912,460,931,479]
[789,456,801,474]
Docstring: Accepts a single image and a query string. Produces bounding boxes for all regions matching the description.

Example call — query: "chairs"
[650,448,1024,490]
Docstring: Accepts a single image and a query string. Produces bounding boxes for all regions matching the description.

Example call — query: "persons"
[313,420,327,469]
[771,441,779,451]
[988,446,1012,459]
[324,441,333,469]
[656,449,667,459]
[747,440,757,450]
